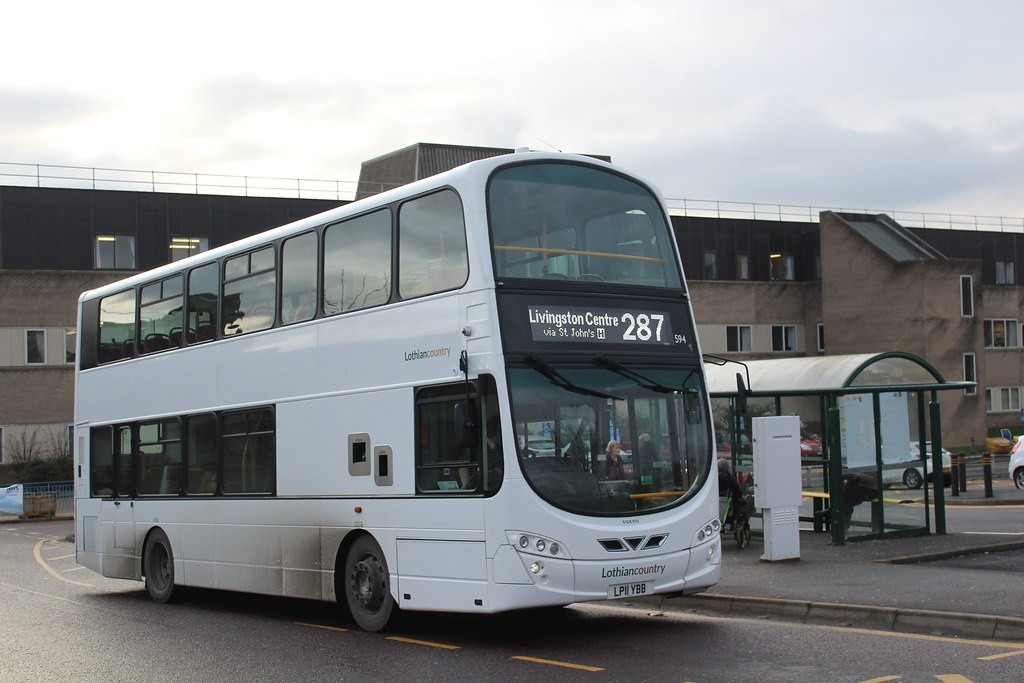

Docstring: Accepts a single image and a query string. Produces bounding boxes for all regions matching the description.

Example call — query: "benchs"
[802,491,915,543]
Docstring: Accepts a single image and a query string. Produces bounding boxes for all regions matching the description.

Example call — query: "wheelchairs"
[719,488,751,549]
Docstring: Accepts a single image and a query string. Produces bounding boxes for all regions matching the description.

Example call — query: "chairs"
[160,463,182,494]
[97,307,276,364]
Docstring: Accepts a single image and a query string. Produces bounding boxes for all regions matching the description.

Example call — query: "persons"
[717,458,752,523]
[816,471,878,529]
[466,415,502,489]
[197,471,218,493]
[606,441,625,480]
[638,433,653,476]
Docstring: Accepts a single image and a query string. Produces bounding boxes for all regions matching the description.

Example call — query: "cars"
[800,438,823,457]
[1007,435,1024,491]
[518,434,566,459]
[880,439,952,490]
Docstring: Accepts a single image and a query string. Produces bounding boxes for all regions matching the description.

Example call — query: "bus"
[71,148,753,632]
